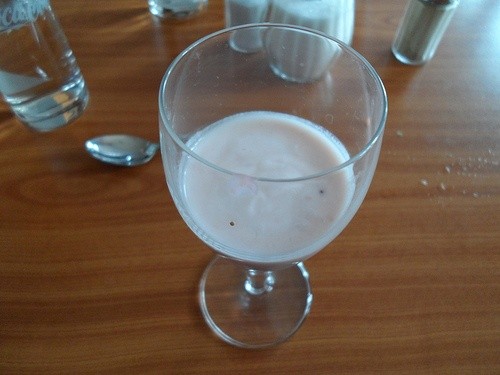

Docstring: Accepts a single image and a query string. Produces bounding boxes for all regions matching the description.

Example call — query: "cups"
[0,1,88,133]
[226,1,357,82]
[391,0,462,64]
[147,1,207,18]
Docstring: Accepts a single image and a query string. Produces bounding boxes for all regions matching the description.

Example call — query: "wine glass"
[160,24,389,350]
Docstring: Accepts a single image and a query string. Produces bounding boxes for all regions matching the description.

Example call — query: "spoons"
[85,135,162,168]
